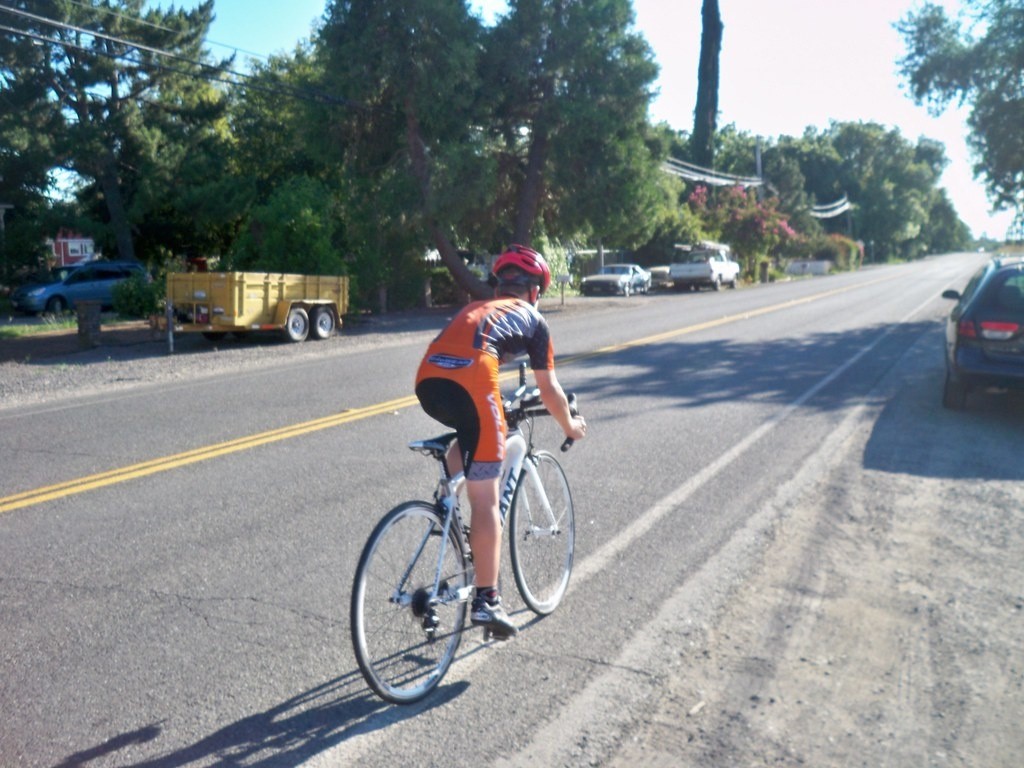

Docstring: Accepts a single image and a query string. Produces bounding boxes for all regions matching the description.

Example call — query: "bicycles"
[351,351,592,706]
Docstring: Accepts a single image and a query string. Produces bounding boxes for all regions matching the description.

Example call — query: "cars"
[581,261,650,299]
[13,261,154,318]
[940,257,1024,416]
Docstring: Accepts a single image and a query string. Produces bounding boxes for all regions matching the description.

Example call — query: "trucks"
[151,268,348,343]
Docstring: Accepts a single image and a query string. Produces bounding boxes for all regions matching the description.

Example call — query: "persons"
[415,242,588,640]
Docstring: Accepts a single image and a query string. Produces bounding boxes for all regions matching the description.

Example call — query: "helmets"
[493,243,551,294]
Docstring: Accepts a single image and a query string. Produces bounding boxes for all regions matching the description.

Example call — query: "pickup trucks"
[664,250,739,295]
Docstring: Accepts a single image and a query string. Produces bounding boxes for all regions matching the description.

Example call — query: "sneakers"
[471,600,519,636]
[432,491,463,530]
